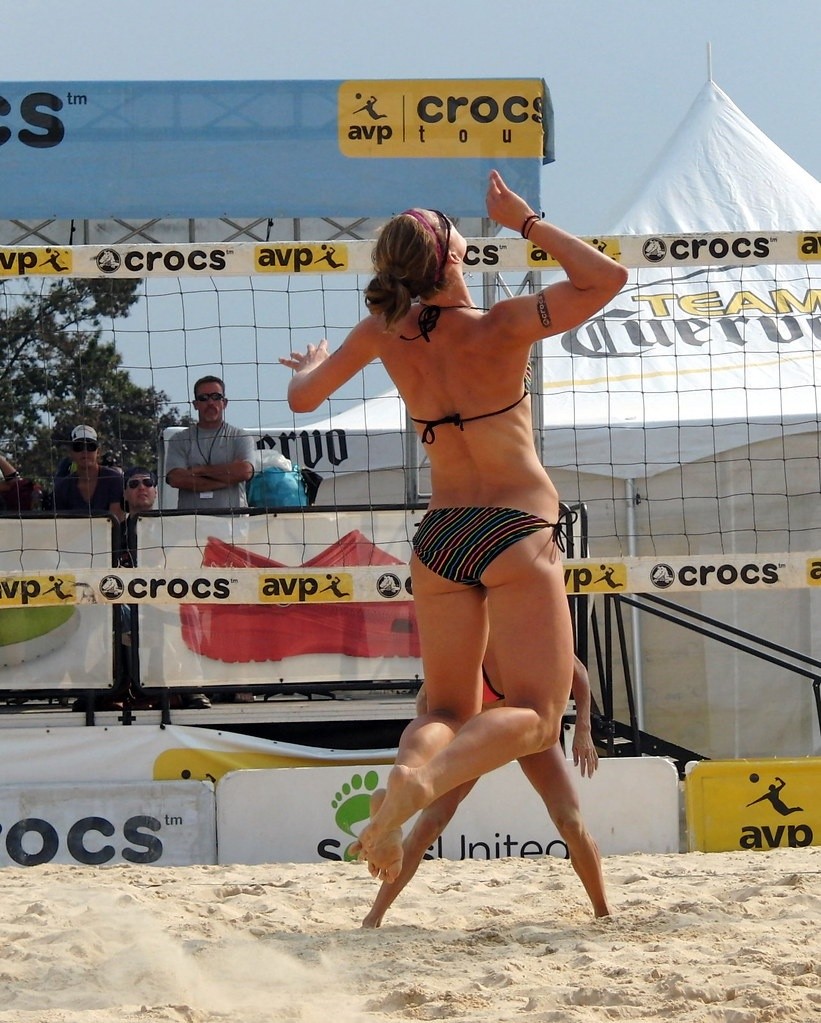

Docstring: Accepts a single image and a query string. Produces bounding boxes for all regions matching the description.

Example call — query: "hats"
[70,425,97,442]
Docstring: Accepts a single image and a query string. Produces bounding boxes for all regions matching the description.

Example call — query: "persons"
[278,168,629,884]
[166,376,255,567]
[362,632,611,929]
[0,425,211,710]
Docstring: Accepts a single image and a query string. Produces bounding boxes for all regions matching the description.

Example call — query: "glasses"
[422,208,453,273]
[71,440,98,453]
[127,479,156,489]
[33,494,44,500]
[194,391,224,403]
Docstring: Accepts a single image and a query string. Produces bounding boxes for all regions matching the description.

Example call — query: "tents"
[152,45,821,761]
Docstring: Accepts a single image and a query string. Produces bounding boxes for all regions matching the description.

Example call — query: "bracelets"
[5,471,19,481]
[521,214,541,239]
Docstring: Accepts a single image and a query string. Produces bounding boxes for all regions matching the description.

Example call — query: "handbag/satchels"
[246,463,309,507]
